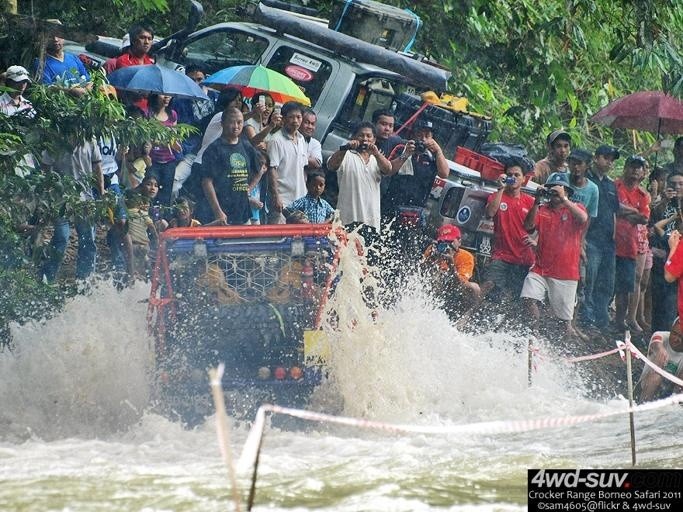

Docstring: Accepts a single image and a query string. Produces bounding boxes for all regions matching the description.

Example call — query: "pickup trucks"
[38,1,536,265]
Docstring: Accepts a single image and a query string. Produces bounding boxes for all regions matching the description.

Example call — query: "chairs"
[191,262,308,306]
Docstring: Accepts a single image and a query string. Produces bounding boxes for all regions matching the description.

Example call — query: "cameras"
[414,138,426,152]
[436,240,458,253]
[537,188,558,197]
[501,174,515,184]
[340,143,368,153]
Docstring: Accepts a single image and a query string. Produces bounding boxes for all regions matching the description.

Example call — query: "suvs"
[145,222,375,424]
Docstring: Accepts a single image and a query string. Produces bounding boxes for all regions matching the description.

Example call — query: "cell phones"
[667,182,675,192]
[259,95,265,106]
[275,108,281,113]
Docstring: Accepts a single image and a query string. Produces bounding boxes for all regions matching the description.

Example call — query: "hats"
[625,155,646,167]
[96,85,118,102]
[596,145,620,159]
[544,172,574,197]
[567,150,593,162]
[6,65,33,84]
[413,118,435,132]
[437,224,461,242]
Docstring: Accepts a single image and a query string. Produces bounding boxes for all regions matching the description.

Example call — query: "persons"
[1,18,683,404]
